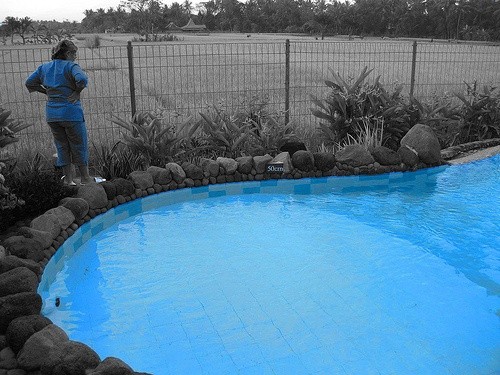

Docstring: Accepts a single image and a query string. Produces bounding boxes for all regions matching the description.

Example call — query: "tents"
[166,17,207,31]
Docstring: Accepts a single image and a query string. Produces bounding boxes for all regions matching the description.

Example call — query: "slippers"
[80,178,106,186]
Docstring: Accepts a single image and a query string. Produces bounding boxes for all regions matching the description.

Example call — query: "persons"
[25,39,107,186]
